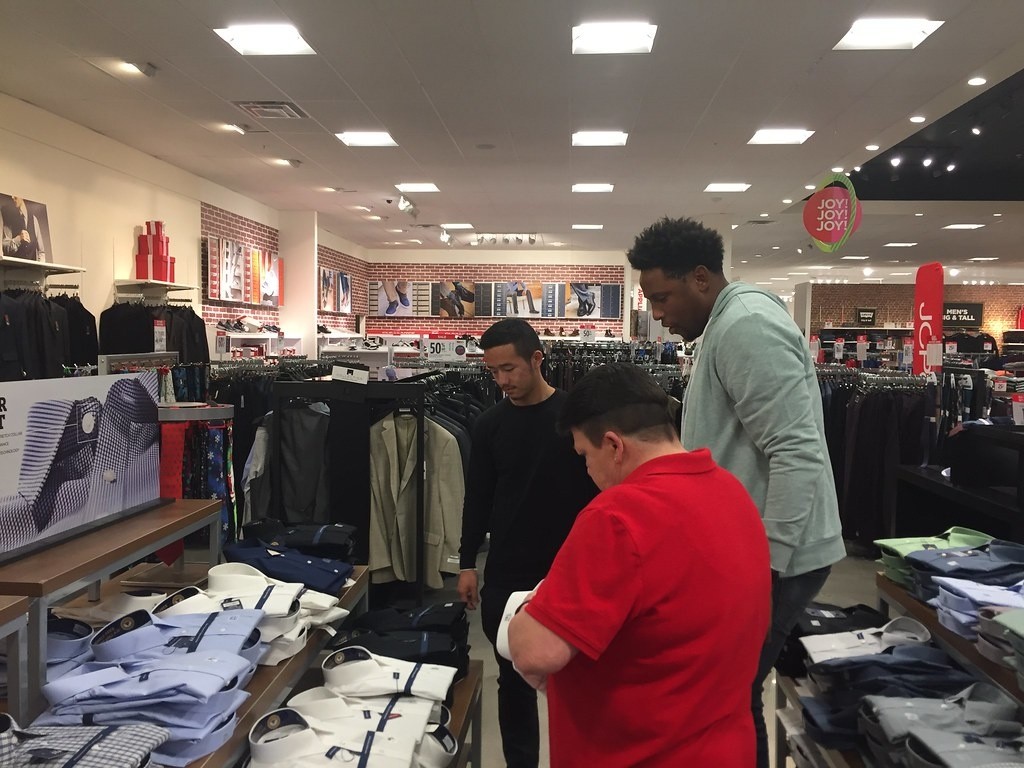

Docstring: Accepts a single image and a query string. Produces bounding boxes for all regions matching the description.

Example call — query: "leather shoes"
[587,293,596,315]
[577,303,587,317]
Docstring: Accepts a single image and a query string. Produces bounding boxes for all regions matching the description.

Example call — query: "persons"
[509,363,774,768]
[506,282,541,314]
[628,219,850,768]
[2,195,46,265]
[261,251,276,301]
[382,281,410,316]
[570,283,596,317]
[322,267,333,308]
[440,282,475,317]
[340,273,350,307]
[454,319,603,768]
[225,240,241,299]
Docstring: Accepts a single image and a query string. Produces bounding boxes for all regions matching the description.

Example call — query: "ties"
[21,214,26,231]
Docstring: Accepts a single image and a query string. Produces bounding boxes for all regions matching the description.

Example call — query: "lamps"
[365,205,374,212]
[418,239,424,245]
[477,234,485,244]
[380,216,390,223]
[489,233,498,245]
[402,228,408,234]
[515,232,524,245]
[233,123,249,136]
[285,158,303,169]
[529,232,537,245]
[334,188,343,194]
[131,61,156,79]
[502,234,511,245]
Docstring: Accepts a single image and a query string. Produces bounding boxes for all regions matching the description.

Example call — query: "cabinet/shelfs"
[819,325,917,355]
[116,277,199,292]
[0,590,30,730]
[368,331,421,355]
[2,256,88,276]
[889,422,1024,539]
[0,499,485,768]
[538,336,623,341]
[1001,328,1024,346]
[772,572,1024,768]
[316,326,367,353]
[424,334,484,355]
[214,325,304,358]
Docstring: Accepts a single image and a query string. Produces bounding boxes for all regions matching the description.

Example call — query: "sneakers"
[385,300,400,316]
[395,286,410,308]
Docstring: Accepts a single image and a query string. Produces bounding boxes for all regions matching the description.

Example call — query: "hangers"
[942,325,990,338]
[2,281,86,305]
[813,352,998,389]
[392,357,494,419]
[543,343,695,391]
[210,355,369,407]
[107,296,197,316]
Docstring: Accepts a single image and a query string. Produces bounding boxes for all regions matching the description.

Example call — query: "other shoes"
[569,329,579,337]
[560,328,566,335]
[514,309,518,314]
[341,297,345,305]
[455,286,475,303]
[458,335,484,350]
[217,320,250,333]
[319,324,332,333]
[448,291,464,315]
[544,329,555,336]
[393,339,427,351]
[344,288,350,306]
[361,339,377,350]
[529,309,539,313]
[263,325,280,332]
[605,329,615,337]
